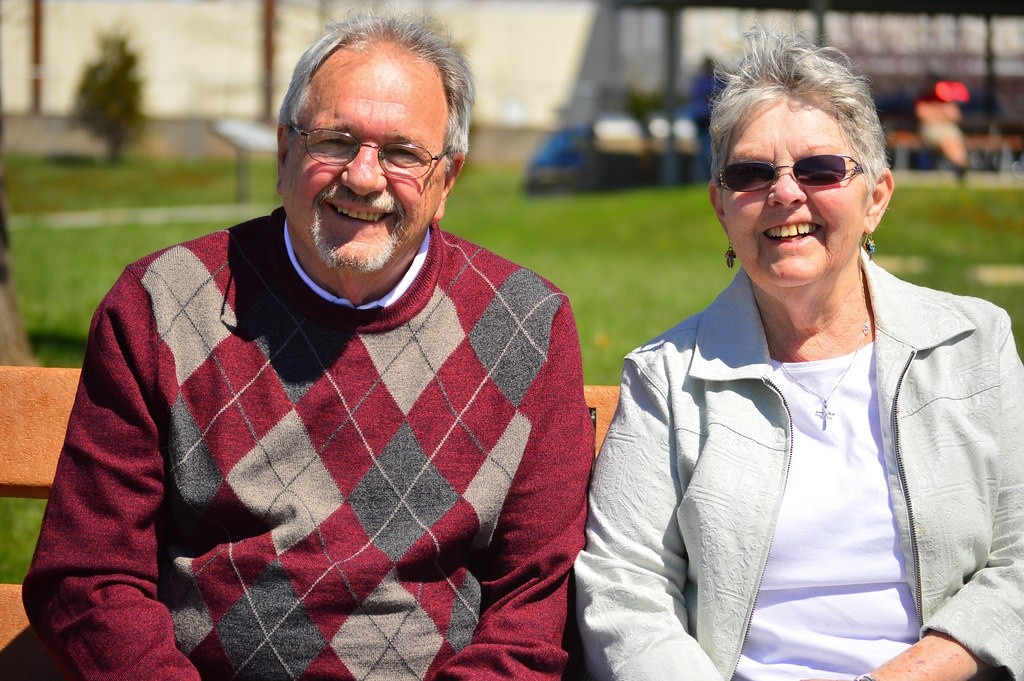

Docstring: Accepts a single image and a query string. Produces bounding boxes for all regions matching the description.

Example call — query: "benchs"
[883,132,1023,174]
[0,367,621,681]
[874,75,1024,174]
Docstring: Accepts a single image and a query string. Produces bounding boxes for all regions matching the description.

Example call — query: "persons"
[21,10,596,681]
[576,21,1024,680]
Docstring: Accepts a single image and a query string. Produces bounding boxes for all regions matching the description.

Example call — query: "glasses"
[291,122,450,180]
[715,155,863,192]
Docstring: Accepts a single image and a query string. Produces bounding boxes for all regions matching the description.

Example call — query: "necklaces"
[772,315,870,431]
